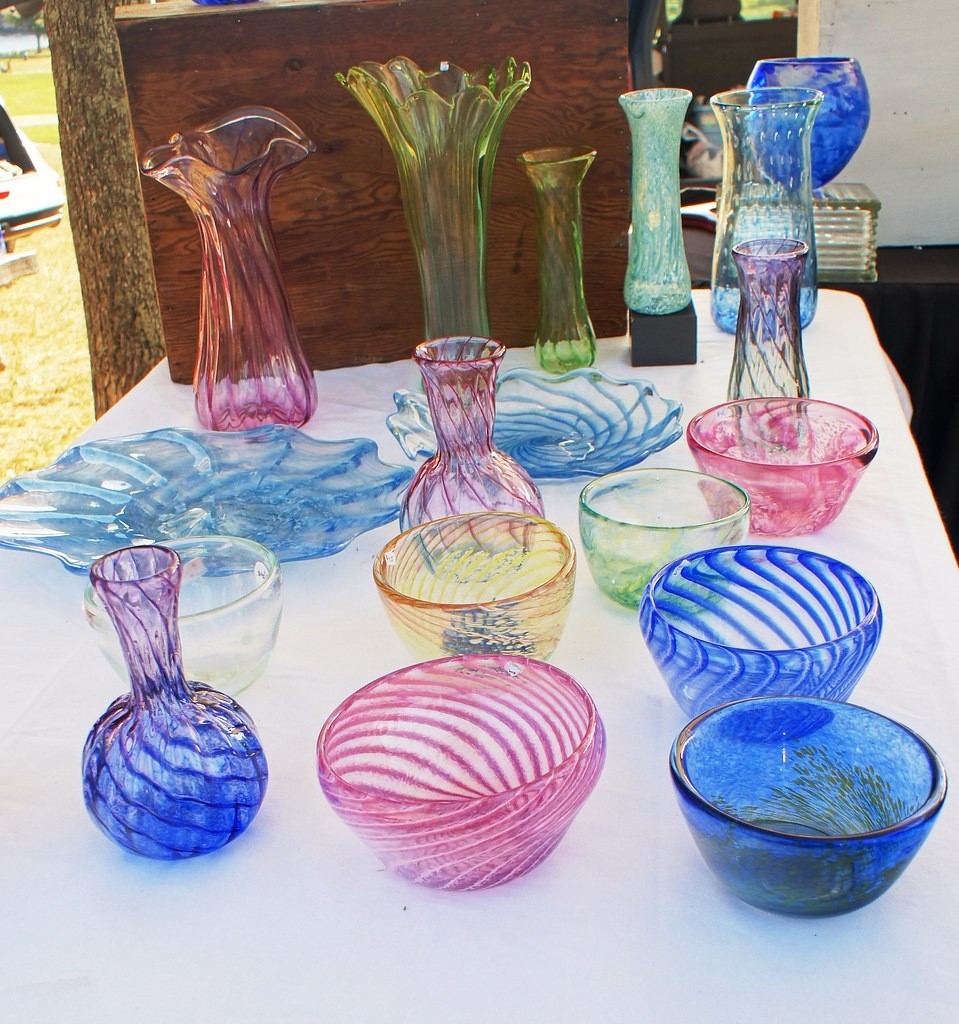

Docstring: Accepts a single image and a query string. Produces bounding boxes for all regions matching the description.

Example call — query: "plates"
[386,369,682,487]
[0,424,414,577]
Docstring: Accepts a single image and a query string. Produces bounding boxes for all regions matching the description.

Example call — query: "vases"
[708,87,821,337]
[519,144,600,372]
[136,106,319,440]
[729,239,811,452]
[80,546,268,859]
[337,55,533,397]
[619,85,694,314]
[398,334,546,577]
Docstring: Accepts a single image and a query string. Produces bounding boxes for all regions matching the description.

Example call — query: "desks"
[0,285,959,1024]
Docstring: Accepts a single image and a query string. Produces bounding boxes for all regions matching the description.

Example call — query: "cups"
[747,55,871,191]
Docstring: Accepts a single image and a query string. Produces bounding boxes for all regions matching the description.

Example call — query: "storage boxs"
[812,182,882,283]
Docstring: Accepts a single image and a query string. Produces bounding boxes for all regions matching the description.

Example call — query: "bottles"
[517,146,601,369]
[396,336,541,584]
[619,87,692,313]
[711,87,816,335]
[81,544,268,860]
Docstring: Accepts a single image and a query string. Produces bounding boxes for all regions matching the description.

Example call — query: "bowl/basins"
[317,654,609,889]
[669,694,947,920]
[373,510,571,667]
[576,466,746,612]
[690,393,877,536]
[83,535,279,704]
[639,543,884,742]
